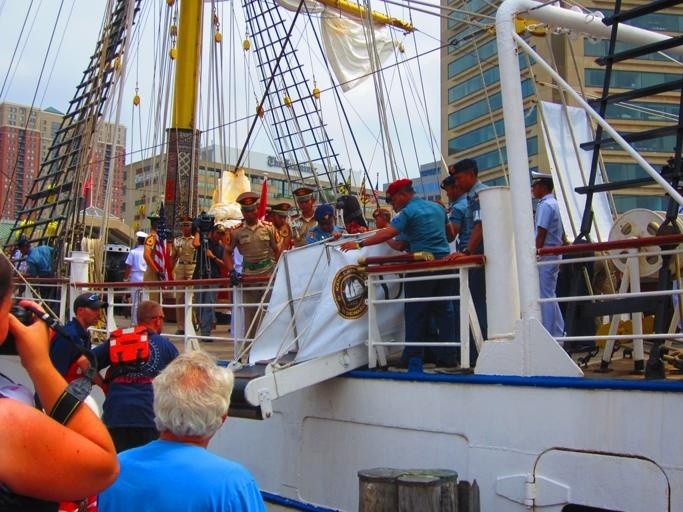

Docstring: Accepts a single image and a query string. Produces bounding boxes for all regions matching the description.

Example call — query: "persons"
[15,238,55,276]
[120,159,613,370]
[0,251,121,511]
[47,292,109,388]
[90,300,182,455]
[95,349,268,512]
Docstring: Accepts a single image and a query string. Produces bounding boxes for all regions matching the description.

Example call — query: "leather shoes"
[178,261,196,264]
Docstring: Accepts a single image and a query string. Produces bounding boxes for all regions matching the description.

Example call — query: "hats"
[314,205,333,220]
[292,188,313,202]
[372,208,389,218]
[235,192,259,211]
[448,159,477,176]
[386,179,412,198]
[147,217,167,225]
[531,170,552,186]
[270,203,290,216]
[136,231,147,238]
[440,176,454,189]
[214,224,225,233]
[73,293,108,312]
[180,217,192,224]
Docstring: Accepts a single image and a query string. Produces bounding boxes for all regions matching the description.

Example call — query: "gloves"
[229,270,241,287]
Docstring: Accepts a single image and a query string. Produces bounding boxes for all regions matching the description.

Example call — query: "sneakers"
[176,330,184,335]
[202,338,212,341]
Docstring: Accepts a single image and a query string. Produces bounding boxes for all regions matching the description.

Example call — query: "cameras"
[10,306,39,325]
